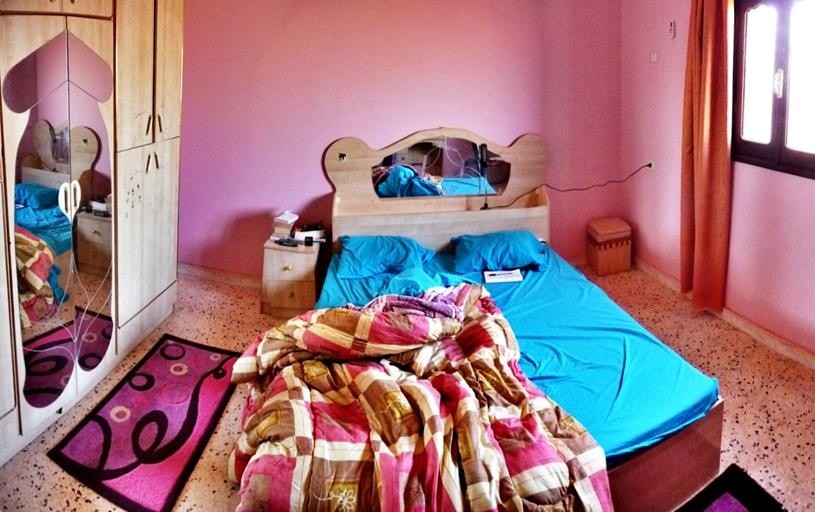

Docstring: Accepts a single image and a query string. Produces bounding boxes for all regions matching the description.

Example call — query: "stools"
[584,217,633,275]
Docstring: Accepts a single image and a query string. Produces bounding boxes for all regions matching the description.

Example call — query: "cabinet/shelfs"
[113,1,184,151]
[3,248,23,468]
[2,12,117,445]
[113,136,182,362]
[0,0,117,24]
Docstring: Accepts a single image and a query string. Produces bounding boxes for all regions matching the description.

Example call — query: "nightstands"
[255,220,330,317]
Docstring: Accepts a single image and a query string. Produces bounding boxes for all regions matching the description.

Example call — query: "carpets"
[43,328,242,512]
[669,458,787,509]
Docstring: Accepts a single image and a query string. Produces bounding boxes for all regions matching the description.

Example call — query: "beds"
[244,126,728,512]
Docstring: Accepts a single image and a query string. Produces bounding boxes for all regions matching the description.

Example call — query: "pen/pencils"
[489,273,513,277]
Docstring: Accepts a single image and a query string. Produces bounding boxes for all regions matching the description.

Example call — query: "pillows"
[334,233,437,282]
[441,227,552,275]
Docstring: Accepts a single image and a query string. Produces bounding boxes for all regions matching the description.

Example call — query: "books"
[268,210,327,245]
[484,268,523,283]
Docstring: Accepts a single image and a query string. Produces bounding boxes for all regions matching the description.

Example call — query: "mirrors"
[4,16,112,409]
[366,134,511,198]
